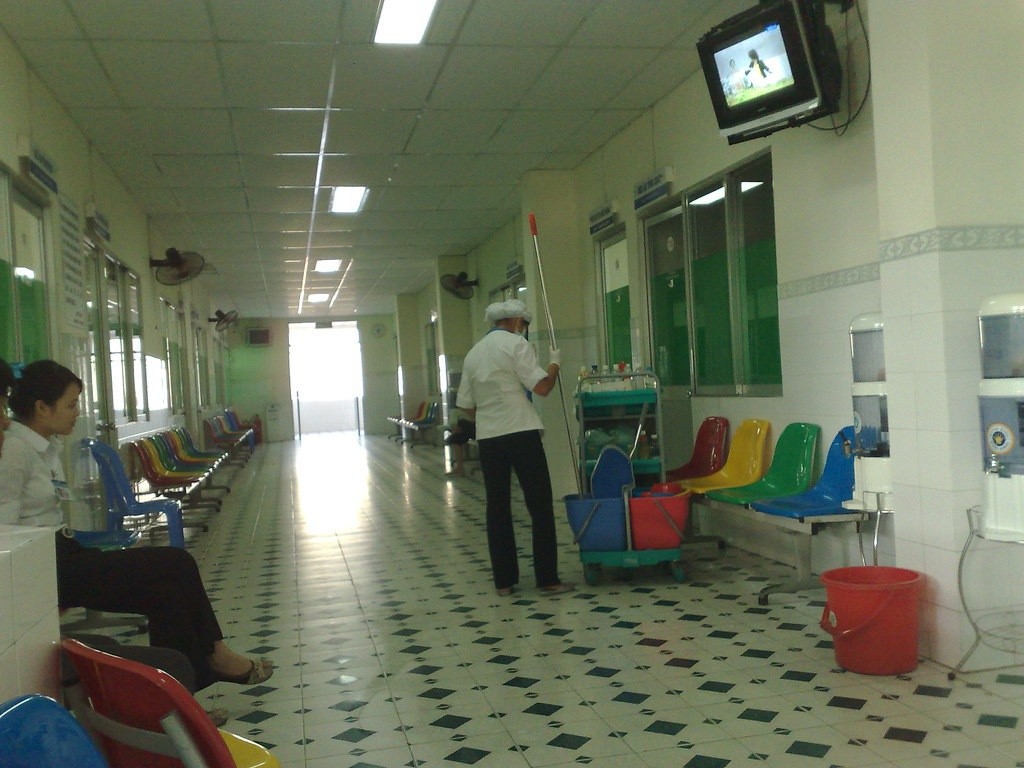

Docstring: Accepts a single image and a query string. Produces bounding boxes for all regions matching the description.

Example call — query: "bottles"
[639,431,649,459]
[623,364,632,381]
[578,366,589,386]
[651,435,657,448]
[612,364,620,382]
[600,364,611,383]
[633,368,644,389]
[589,365,600,385]
[645,367,654,389]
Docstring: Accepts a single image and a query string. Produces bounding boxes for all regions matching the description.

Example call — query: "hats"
[484,299,534,324]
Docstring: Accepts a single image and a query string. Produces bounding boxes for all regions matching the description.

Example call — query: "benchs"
[663,415,878,606]
[127,425,230,531]
[205,416,250,467]
[59,526,149,633]
[388,402,440,449]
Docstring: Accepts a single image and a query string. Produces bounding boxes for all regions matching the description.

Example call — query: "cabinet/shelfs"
[574,372,682,580]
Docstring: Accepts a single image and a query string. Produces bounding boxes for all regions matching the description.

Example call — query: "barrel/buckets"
[820,566,922,675]
[629,482,692,550]
[562,490,629,551]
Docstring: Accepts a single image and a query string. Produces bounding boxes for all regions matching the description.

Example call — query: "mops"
[525,209,586,501]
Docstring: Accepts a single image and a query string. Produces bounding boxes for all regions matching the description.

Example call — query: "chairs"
[0,637,282,768]
[223,408,257,451]
[83,436,186,548]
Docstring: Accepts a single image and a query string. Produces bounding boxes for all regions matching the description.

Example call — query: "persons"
[729,48,772,90]
[1,359,275,691]
[457,298,578,595]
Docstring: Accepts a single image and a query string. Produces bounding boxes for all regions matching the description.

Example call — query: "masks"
[514,321,526,337]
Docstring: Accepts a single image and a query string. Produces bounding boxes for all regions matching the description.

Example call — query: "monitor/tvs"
[695,0,842,138]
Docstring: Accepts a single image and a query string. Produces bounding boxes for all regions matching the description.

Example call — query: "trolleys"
[574,369,683,585]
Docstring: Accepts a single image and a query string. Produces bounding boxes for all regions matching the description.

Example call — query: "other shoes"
[497,586,513,596]
[207,657,272,685]
[543,584,573,594]
[445,471,464,479]
[207,706,230,726]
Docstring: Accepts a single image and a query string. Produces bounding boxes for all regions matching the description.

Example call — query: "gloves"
[549,346,561,367]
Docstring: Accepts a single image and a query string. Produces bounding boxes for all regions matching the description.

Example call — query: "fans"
[208,308,238,331]
[440,272,479,300]
[150,247,205,286]
